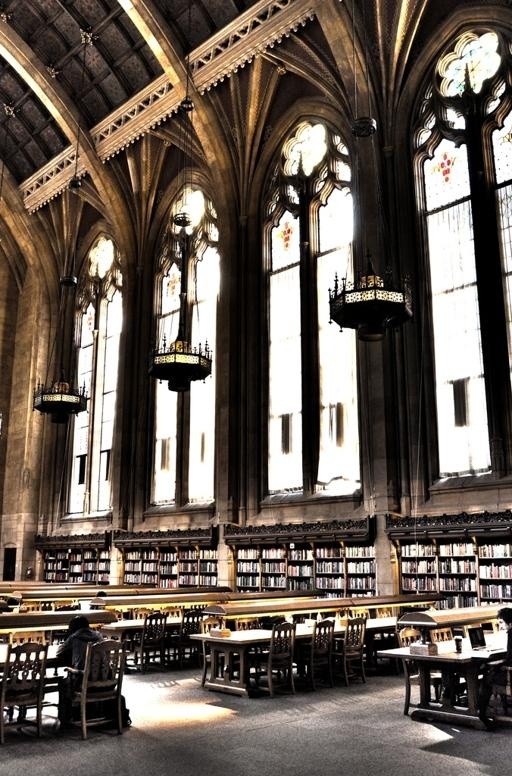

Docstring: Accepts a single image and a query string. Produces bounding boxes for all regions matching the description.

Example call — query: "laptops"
[467,627,505,651]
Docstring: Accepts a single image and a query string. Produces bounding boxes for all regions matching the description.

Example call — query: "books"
[402,544,512,612]
[42,548,111,584]
[123,546,218,589]
[235,545,378,599]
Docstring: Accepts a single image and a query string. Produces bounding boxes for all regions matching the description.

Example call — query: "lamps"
[329,0,413,334]
[150,1,212,392]
[30,30,89,416]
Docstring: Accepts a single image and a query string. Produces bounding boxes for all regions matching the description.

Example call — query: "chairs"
[64,637,127,740]
[1,577,511,725]
[2,641,50,742]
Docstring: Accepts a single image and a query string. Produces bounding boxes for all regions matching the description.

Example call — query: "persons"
[475,607,511,722]
[54,615,112,736]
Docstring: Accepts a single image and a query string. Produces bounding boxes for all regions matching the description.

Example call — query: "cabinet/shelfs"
[385,509,512,611]
[222,515,377,597]
[113,522,218,587]
[33,530,110,585]
